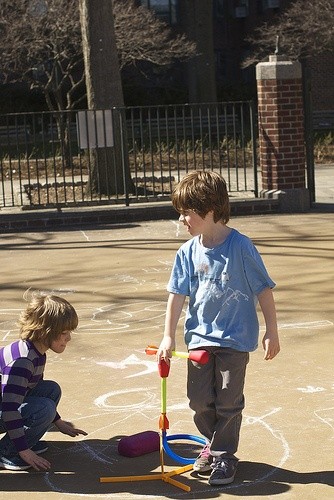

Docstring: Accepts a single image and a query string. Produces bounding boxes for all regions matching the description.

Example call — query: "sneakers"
[193,445,213,472]
[209,458,238,485]
[31,442,48,454]
[0,455,31,470]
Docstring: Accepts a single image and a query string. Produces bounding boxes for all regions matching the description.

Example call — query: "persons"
[0,295,88,471]
[155,169,282,485]
